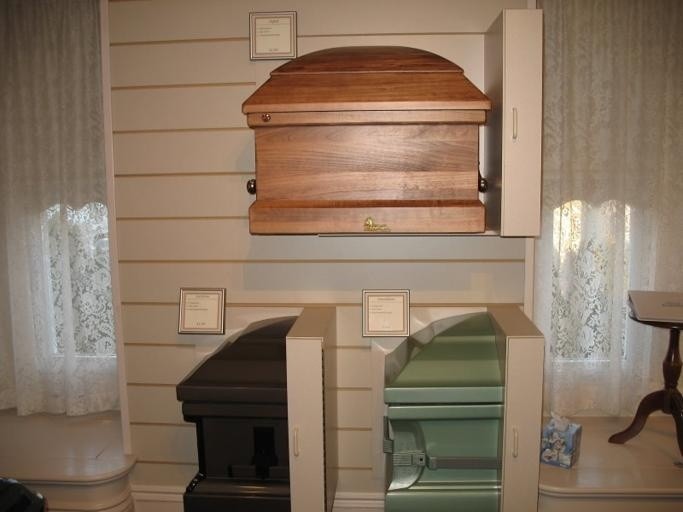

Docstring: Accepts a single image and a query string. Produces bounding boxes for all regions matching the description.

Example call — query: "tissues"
[540,411,583,469]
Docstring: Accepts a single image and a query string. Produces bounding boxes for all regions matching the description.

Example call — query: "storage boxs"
[242,47,493,235]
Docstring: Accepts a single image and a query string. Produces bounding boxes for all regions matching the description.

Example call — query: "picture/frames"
[178,287,225,335]
[248,10,298,61]
[362,289,410,337]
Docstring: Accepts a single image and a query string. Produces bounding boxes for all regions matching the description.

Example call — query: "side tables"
[608,309,683,457]
